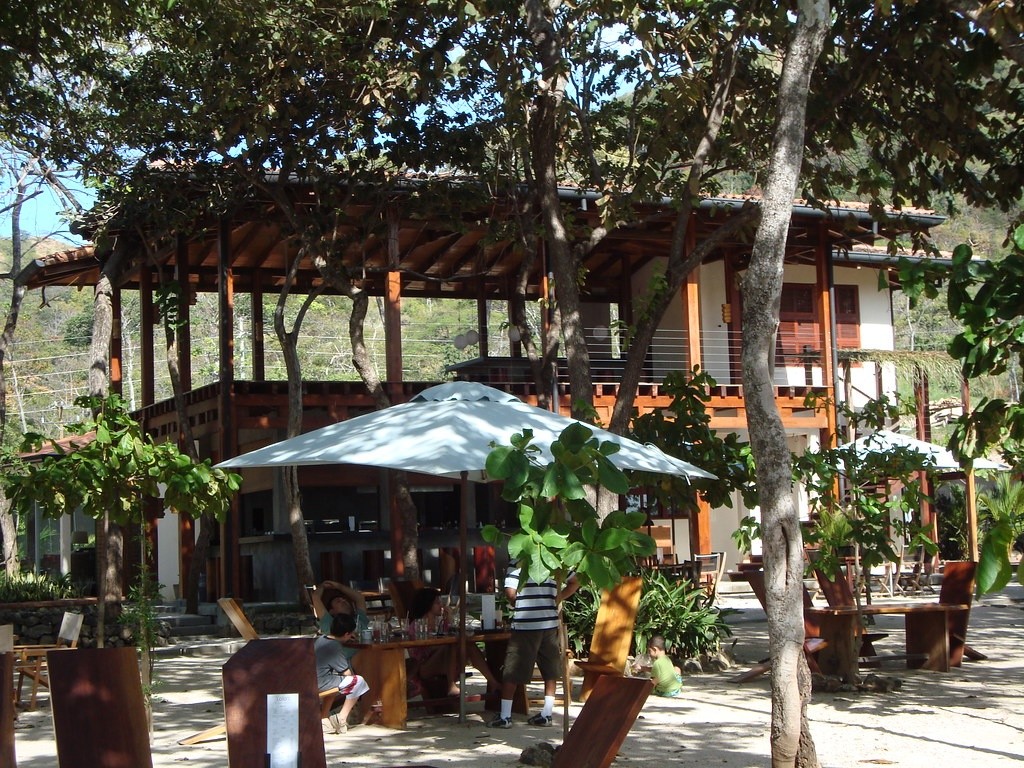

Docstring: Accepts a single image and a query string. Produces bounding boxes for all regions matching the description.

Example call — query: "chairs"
[0,543,987,768]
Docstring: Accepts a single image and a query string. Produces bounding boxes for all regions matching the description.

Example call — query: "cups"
[480,615,484,630]
[495,610,503,628]
[401,619,409,640]
[354,620,361,641]
[448,624,457,634]
[482,595,495,629]
[502,620,506,630]
[373,621,381,641]
[415,619,421,637]
[381,622,389,642]
[436,616,444,637]
[422,619,428,636]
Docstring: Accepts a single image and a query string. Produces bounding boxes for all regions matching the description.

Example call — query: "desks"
[803,600,969,682]
[342,629,530,730]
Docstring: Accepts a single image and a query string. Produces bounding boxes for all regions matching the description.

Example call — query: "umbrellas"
[210,381,720,726]
[788,428,1009,596]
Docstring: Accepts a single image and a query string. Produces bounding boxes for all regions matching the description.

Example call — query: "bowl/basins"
[465,694,481,702]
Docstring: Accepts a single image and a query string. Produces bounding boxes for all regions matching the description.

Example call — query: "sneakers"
[322,715,349,735]
[528,712,552,727]
[487,713,513,729]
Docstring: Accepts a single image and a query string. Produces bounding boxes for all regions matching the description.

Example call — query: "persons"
[407,588,503,696]
[314,613,369,735]
[311,581,370,715]
[646,635,682,698]
[486,525,581,728]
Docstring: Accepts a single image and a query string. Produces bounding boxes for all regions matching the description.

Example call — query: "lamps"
[592,325,608,341]
[465,301,479,345]
[453,299,467,349]
[507,325,521,341]
